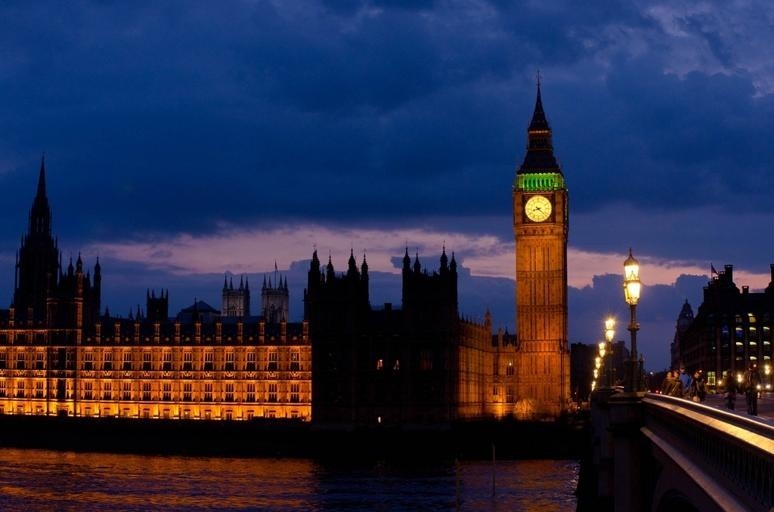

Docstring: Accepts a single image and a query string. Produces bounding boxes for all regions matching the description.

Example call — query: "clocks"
[524,194,552,223]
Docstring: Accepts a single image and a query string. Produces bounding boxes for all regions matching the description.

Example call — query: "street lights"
[738,374,743,394]
[624,247,645,392]
[765,365,771,392]
[591,316,615,394]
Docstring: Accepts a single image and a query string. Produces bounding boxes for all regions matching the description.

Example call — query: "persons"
[725,369,740,410]
[662,364,707,403]
[742,365,762,416]
